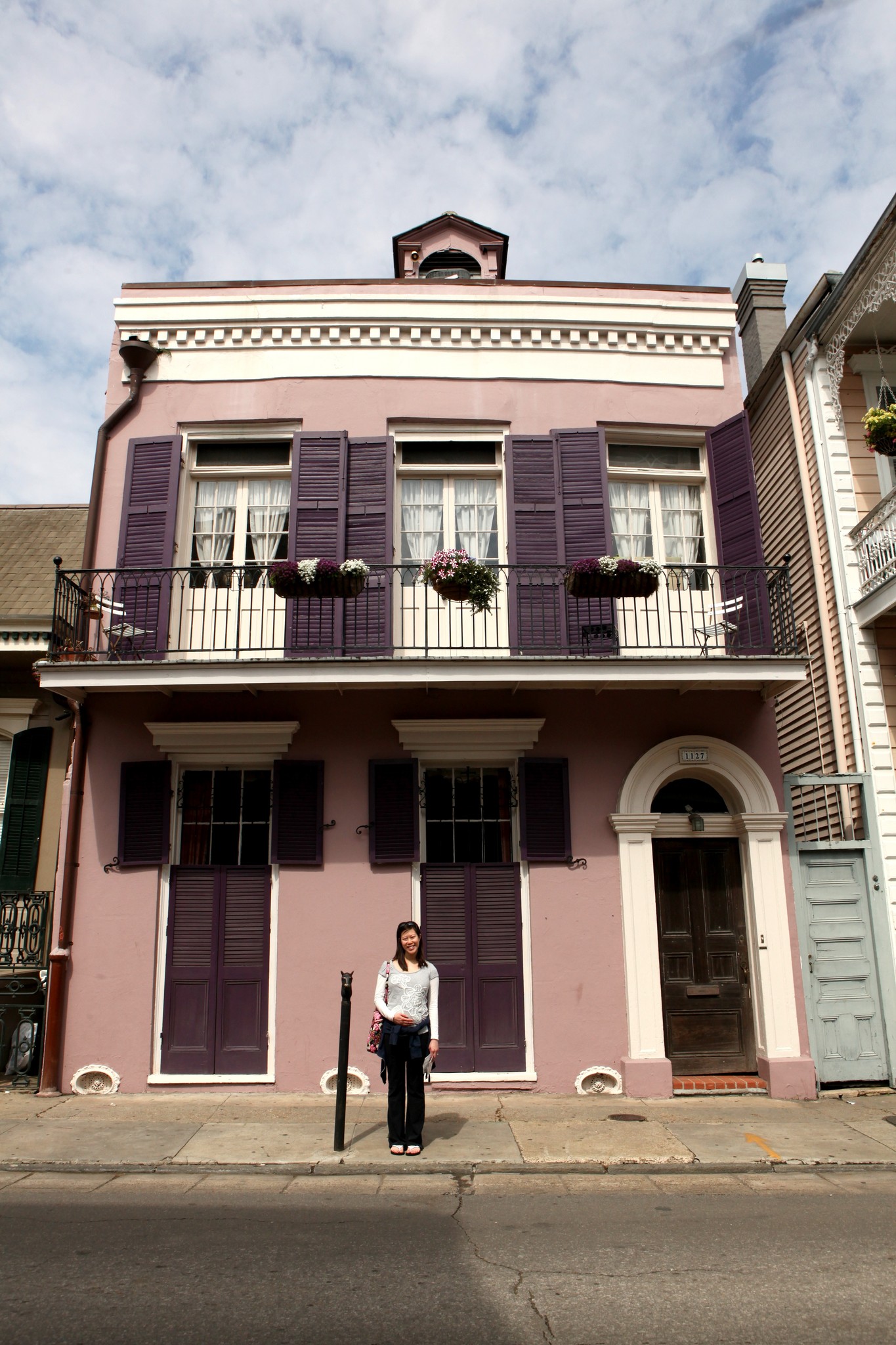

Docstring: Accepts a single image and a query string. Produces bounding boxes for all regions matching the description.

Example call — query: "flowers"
[570,557,662,577]
[861,402,896,454]
[269,557,370,584]
[413,548,500,617]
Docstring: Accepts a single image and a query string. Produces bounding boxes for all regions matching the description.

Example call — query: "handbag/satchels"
[367,1006,384,1054]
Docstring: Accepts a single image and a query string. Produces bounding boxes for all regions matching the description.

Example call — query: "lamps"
[688,813,705,832]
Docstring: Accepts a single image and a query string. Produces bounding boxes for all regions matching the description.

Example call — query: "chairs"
[94,595,154,660]
[690,597,744,655]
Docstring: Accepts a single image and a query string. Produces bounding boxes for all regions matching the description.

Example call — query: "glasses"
[397,921,416,928]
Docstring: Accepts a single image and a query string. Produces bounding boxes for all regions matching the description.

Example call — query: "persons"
[374,920,440,1157]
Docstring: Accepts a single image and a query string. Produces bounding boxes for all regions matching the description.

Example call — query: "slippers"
[405,1145,421,1156]
[390,1144,405,1155]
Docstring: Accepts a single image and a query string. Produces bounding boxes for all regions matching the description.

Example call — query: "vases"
[872,429,896,457]
[433,576,474,602]
[568,573,658,598]
[276,575,363,599]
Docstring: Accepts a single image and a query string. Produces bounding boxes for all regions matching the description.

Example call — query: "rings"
[436,1053,438,1055]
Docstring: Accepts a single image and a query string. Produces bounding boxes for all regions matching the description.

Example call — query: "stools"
[583,623,619,658]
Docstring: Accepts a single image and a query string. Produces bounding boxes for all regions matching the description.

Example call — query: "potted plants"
[81,591,106,619]
[57,639,98,662]
